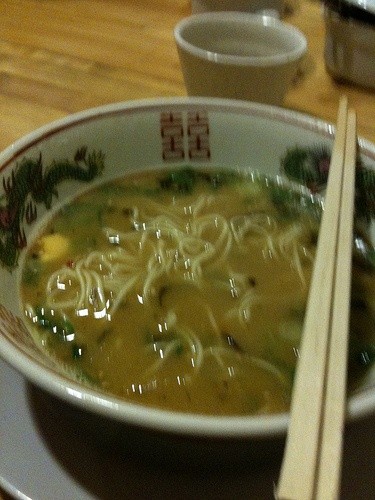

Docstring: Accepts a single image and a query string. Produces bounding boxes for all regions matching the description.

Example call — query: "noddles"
[43,188,310,382]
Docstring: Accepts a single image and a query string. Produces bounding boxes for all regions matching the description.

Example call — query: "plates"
[2,357,374,500]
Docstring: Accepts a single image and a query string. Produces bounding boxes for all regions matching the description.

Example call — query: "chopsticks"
[275,97,359,500]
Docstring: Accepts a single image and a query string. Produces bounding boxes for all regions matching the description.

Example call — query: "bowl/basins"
[2,95,375,446]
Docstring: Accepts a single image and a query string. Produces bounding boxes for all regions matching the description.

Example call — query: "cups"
[321,0,375,93]
[174,11,307,114]
